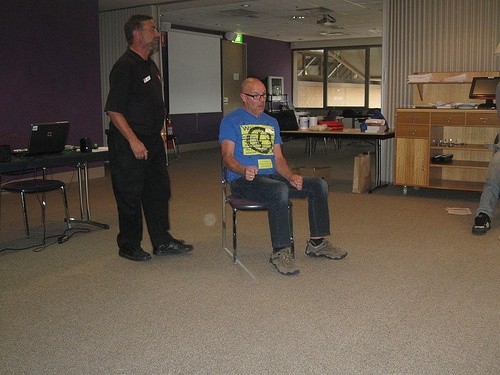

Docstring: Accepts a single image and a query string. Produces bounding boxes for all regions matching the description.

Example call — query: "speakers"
[80,138,92,153]
[225,32,237,41]
[160,22,171,32]
[0,144,12,162]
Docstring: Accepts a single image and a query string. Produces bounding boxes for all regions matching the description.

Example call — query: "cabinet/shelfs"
[393,108,500,196]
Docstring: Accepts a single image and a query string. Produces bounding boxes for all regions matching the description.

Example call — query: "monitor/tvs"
[468,76,499,110]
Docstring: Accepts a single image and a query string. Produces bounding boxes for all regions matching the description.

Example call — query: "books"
[318,118,388,134]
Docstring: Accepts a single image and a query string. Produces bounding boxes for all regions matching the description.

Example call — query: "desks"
[280,127,395,194]
[0,147,110,230]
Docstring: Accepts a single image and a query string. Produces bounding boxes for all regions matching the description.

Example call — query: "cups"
[80,137,92,153]
[0,145,12,161]
[309,117,317,129]
[300,117,307,129]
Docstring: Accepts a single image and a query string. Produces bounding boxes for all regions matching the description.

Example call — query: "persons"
[103,14,194,263]
[219,76,348,277]
[470,79,500,235]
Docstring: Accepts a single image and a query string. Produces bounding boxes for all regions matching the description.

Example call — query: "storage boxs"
[290,166,332,188]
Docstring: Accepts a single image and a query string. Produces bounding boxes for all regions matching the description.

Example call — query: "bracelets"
[250,176,252,178]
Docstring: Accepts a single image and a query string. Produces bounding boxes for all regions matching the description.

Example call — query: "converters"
[58,236,68,244]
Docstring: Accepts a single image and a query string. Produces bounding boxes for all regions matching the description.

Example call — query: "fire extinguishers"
[166,118,175,149]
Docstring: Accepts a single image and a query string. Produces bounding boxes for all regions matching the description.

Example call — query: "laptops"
[12,121,70,155]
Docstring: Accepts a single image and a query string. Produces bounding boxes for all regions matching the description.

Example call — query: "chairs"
[0,133,73,239]
[220,142,295,267]
[304,109,353,155]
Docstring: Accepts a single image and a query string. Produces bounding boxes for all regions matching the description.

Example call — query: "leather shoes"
[119,246,151,261]
[244,92,268,100]
[153,238,193,256]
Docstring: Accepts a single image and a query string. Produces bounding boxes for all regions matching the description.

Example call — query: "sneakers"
[305,237,347,260]
[270,247,299,276]
[471,210,490,235]
